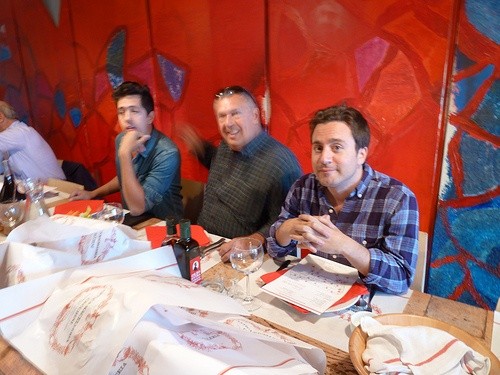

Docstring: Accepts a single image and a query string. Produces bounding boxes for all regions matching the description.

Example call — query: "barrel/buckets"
[0,192,32,236]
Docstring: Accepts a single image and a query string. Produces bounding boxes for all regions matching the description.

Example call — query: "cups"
[102,203,124,224]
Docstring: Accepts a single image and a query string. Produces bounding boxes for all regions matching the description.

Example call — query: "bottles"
[160,216,203,284]
[22,177,51,224]
[0,159,26,201]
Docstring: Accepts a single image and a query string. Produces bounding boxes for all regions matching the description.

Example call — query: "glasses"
[215,86,257,104]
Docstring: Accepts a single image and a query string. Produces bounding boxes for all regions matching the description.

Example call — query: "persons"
[0,101,66,183]
[69,81,185,220]
[268,104,419,294]
[183,85,304,263]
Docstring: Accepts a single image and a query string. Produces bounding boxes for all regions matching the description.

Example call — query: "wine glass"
[230,238,265,311]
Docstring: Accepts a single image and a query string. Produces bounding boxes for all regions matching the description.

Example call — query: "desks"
[0,176,500,375]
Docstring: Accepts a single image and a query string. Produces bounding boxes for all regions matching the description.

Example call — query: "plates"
[204,230,211,240]
[47,198,107,217]
[324,295,362,313]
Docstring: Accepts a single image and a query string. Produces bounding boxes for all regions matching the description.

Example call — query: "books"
[45,189,70,207]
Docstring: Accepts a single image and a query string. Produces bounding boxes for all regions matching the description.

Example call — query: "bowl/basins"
[348,313,500,375]
